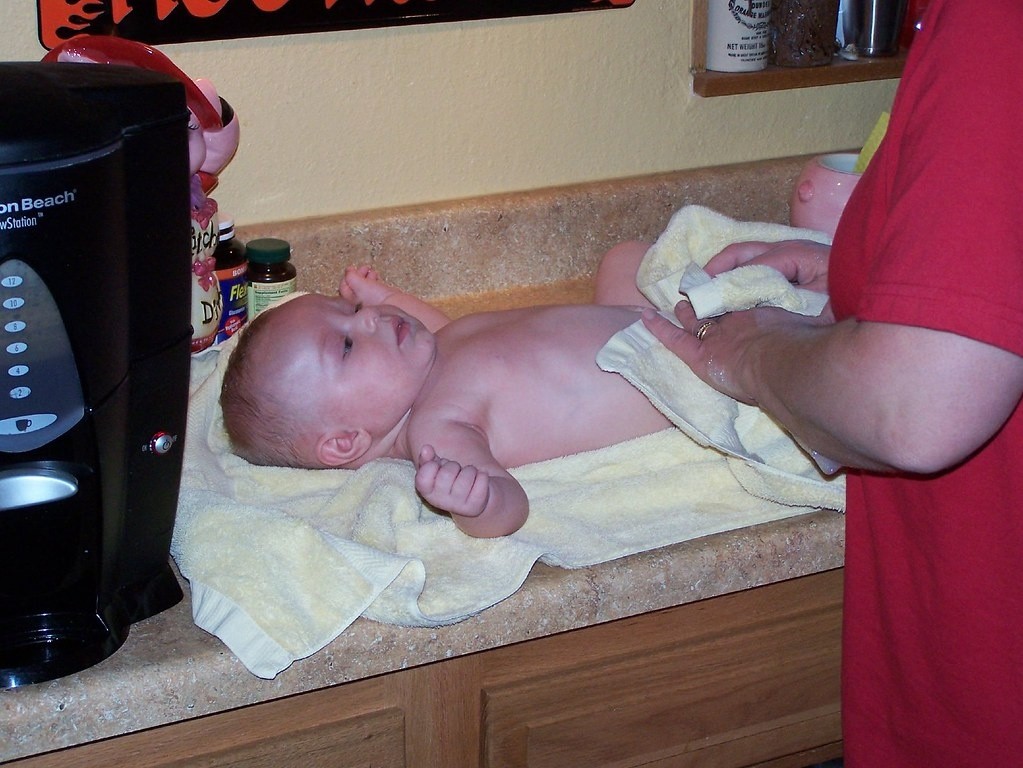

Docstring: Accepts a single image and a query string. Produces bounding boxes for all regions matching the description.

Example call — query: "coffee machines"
[0,60,193,689]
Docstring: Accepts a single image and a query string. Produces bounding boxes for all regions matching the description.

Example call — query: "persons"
[642,0,1023,768]
[219,242,842,538]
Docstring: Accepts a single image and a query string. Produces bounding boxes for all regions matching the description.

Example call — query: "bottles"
[212,219,249,337]
[245,238,296,323]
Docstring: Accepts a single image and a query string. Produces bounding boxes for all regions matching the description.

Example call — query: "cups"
[792,152,862,234]
[705,0,772,71]
[775,0,841,68]
[857,0,908,57]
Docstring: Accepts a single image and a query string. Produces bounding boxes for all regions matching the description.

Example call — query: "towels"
[170,202,846,682]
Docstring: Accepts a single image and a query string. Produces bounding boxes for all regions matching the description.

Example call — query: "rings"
[696,322,717,340]
